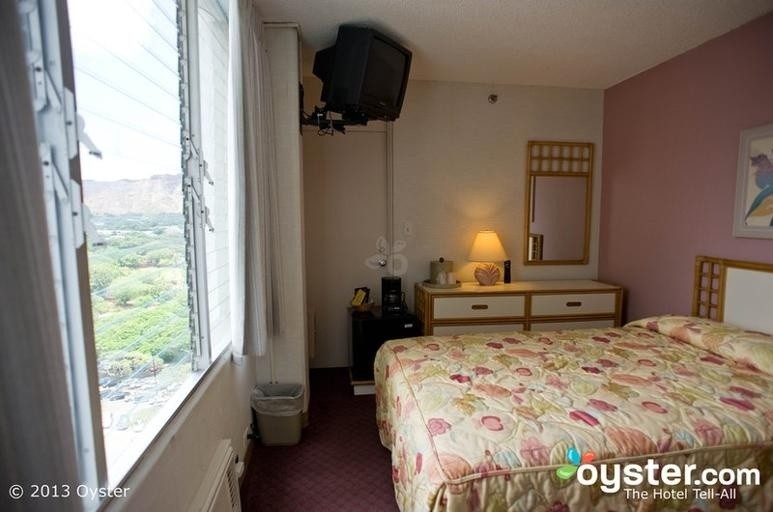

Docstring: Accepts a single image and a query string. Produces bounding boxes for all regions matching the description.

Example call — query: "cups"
[448,272,457,284]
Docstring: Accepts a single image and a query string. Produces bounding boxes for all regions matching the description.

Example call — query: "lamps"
[465,230,512,288]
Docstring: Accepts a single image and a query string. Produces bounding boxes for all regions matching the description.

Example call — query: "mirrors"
[523,140,595,266]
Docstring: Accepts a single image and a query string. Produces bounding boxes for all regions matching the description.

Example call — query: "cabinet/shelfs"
[413,279,624,333]
[341,307,414,394]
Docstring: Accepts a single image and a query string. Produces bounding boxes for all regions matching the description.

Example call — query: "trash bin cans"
[252,383,305,446]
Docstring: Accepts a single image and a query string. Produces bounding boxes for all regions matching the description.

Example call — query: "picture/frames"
[731,122,773,241]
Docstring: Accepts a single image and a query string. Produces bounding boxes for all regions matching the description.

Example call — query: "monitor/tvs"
[312,23,412,123]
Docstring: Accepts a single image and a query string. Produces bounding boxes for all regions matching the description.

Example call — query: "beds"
[371,253,772,512]
